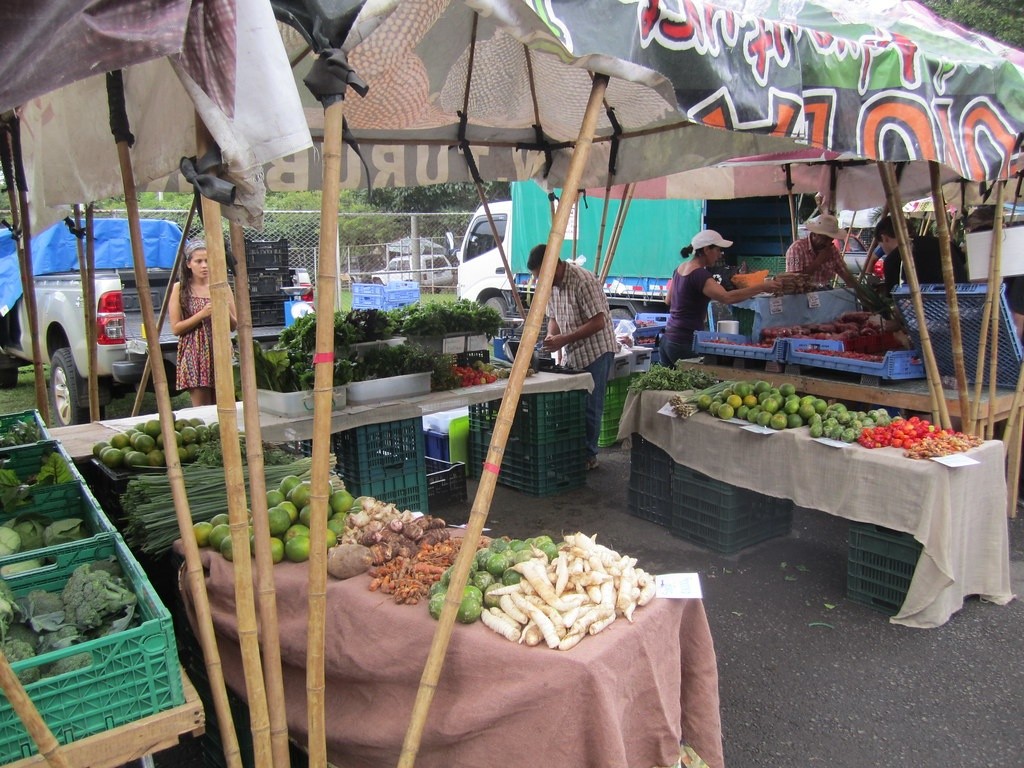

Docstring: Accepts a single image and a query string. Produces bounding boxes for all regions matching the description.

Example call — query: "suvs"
[253,267,314,312]
[370,253,454,294]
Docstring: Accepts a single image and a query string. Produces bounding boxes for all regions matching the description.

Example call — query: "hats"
[691,230,733,256]
[806,214,847,240]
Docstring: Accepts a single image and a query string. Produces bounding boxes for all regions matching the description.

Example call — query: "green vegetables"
[626,362,718,394]
[190,436,295,465]
[0,416,83,515]
[276,296,506,352]
[233,336,461,390]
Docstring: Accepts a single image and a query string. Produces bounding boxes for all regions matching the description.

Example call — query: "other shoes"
[586,454,599,471]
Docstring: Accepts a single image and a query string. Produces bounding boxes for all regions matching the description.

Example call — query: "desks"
[43,370,596,466]
[173,502,723,768]
[677,357,1024,519]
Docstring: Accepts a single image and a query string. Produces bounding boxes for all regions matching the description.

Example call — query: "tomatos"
[858,416,955,448]
[448,367,496,386]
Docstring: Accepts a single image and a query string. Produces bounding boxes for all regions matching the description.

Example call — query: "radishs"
[480,534,657,653]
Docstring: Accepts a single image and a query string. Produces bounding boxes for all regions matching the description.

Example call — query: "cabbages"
[0,513,93,578]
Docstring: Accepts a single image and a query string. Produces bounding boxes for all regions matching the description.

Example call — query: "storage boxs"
[396,451,466,511]
[0,478,117,578]
[469,388,587,445]
[736,255,786,279]
[337,470,429,514]
[301,415,426,478]
[0,531,185,766]
[599,313,793,554]
[254,384,347,418]
[347,371,433,404]
[350,281,420,312]
[425,429,450,463]
[844,526,923,617]
[469,429,588,499]
[0,439,83,493]
[852,521,925,549]
[227,236,297,326]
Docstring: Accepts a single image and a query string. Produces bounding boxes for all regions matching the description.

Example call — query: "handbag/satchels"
[613,319,636,349]
[731,270,768,289]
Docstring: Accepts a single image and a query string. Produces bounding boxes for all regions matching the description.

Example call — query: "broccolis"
[0,563,139,687]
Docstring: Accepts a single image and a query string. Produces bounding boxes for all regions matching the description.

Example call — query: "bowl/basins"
[498,317,525,329]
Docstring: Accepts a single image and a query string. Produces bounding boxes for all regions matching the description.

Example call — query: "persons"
[786,213,857,288]
[527,243,620,470]
[658,230,783,369]
[962,206,1024,343]
[865,217,969,332]
[866,248,885,275]
[169,239,237,407]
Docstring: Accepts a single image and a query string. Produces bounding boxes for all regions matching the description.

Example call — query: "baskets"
[457,350,490,370]
[0,409,185,766]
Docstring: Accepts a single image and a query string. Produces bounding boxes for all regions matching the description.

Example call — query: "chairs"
[0,408,52,448]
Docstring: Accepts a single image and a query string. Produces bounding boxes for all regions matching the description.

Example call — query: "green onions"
[670,380,734,418]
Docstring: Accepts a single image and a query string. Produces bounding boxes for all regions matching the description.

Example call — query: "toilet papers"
[716,320,739,335]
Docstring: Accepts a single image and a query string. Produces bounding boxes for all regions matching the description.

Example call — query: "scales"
[493,316,536,371]
[280,286,315,327]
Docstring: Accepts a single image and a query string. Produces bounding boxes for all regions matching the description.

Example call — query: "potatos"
[701,313,900,363]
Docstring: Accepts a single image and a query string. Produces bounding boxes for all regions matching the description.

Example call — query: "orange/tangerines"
[93,414,220,469]
[194,476,352,563]
[427,529,564,623]
[698,381,901,444]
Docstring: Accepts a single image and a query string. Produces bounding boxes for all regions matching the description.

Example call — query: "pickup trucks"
[0,217,240,428]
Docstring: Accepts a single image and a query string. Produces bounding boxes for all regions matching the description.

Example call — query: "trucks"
[442,176,793,346]
